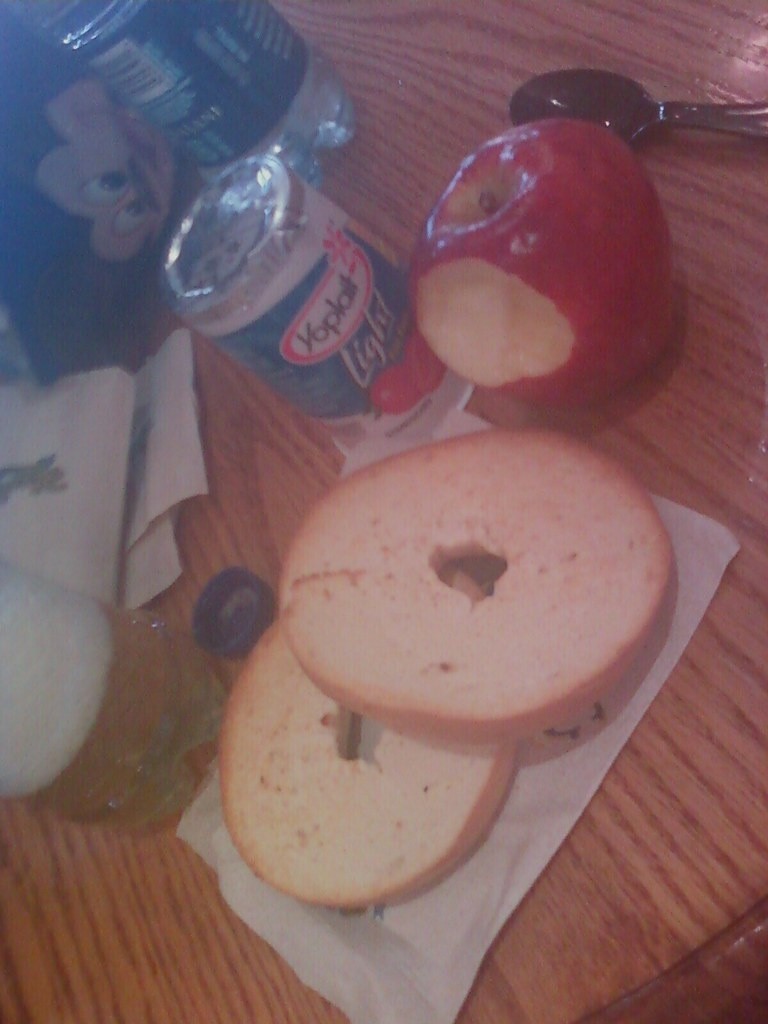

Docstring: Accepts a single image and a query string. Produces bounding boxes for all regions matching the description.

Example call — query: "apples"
[404,115,679,406]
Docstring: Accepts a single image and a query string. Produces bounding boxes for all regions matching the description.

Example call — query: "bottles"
[12,0,356,192]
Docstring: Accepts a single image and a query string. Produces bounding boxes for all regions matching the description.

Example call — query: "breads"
[278,429,673,763]
[218,623,519,907]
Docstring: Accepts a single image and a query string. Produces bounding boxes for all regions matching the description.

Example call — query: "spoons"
[507,70,768,151]
